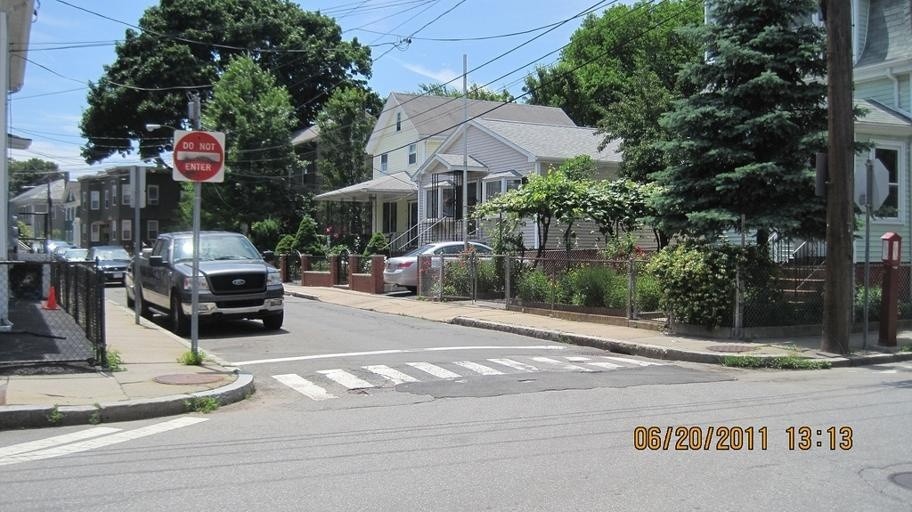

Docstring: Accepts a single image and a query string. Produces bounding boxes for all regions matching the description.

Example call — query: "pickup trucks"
[123,229,285,334]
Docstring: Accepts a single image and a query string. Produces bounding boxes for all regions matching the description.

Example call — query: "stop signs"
[173,129,226,183]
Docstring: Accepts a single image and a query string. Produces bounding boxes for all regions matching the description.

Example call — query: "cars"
[45,239,89,266]
[85,245,131,286]
[383,240,543,295]
